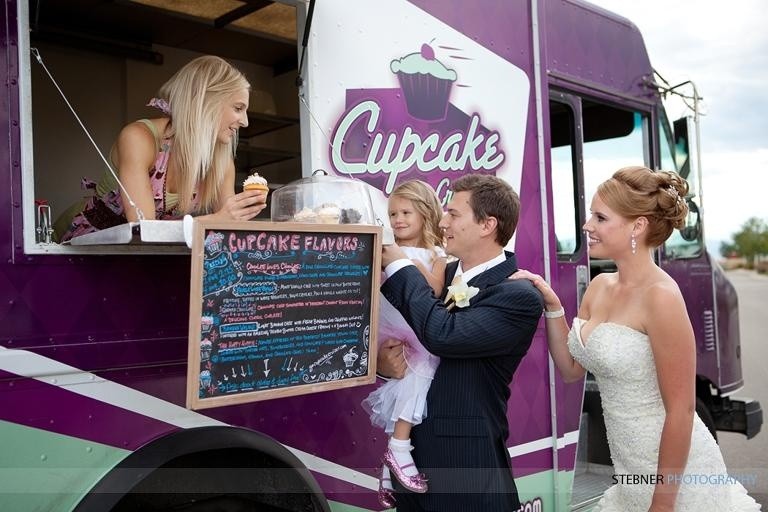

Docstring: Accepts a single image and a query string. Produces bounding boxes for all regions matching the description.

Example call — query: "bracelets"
[543,306,565,319]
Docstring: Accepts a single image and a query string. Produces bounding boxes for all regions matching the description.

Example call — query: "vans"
[1,1,762,510]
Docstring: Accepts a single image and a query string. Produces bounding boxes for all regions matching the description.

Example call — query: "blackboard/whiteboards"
[186,220,382,410]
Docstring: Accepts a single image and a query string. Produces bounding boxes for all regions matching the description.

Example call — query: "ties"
[443,273,463,304]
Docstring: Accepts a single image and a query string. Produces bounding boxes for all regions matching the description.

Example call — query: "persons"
[508,163,763,511]
[374,173,543,512]
[50,54,267,245]
[360,179,448,509]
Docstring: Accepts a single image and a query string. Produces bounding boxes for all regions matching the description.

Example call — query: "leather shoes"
[378,458,398,510]
[380,446,429,495]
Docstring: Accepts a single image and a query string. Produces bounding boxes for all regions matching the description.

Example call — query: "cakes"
[293,202,362,224]
[243,172,269,206]
[390,37,457,120]
